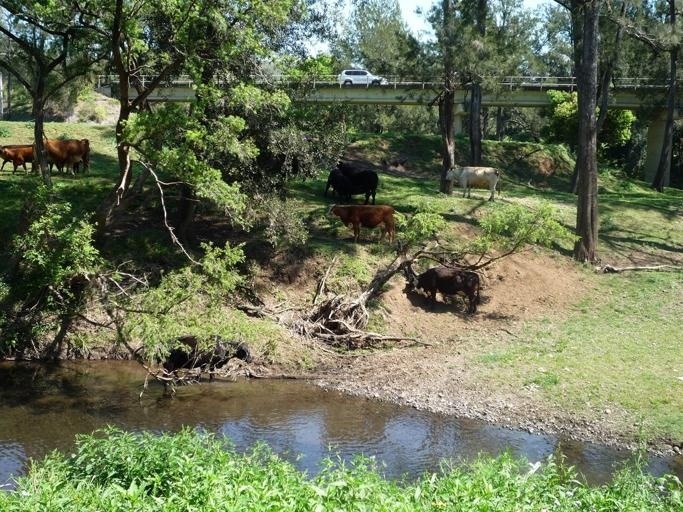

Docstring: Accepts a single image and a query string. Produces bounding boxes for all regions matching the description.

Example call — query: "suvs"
[337,68,390,87]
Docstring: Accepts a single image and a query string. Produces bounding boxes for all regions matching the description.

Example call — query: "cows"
[323,161,378,205]
[325,203,396,246]
[1,138,91,176]
[409,267,480,314]
[445,165,501,202]
